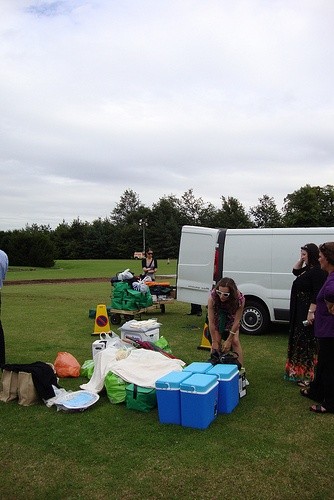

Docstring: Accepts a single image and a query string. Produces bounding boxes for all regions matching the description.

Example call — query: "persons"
[142,248,157,280]
[0,250,9,365]
[207,278,249,385]
[188,303,202,316]
[299,242,334,414]
[284,243,329,386]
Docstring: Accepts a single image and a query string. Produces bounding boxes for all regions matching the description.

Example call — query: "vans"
[134,252,143,260]
[176,225,334,336]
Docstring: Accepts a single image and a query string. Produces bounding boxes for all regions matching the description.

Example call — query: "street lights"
[139,218,149,256]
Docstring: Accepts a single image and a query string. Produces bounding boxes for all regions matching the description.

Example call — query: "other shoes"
[298,381,310,386]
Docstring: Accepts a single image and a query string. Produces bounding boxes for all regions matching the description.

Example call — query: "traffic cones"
[90,304,112,336]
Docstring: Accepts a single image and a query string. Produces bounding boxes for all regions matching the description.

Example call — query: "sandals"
[309,404,330,413]
[300,389,318,399]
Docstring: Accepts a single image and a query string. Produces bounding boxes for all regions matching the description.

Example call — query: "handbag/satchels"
[139,274,155,281]
[207,349,242,371]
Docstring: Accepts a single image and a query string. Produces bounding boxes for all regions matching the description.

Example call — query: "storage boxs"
[155,361,246,429]
[120,329,159,345]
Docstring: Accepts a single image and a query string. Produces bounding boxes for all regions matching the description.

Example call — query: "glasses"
[146,252,153,255]
[320,244,334,254]
[216,290,230,297]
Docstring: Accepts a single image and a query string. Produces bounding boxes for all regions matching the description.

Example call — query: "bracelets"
[230,331,236,334]
[309,310,315,312]
[299,259,303,262]
